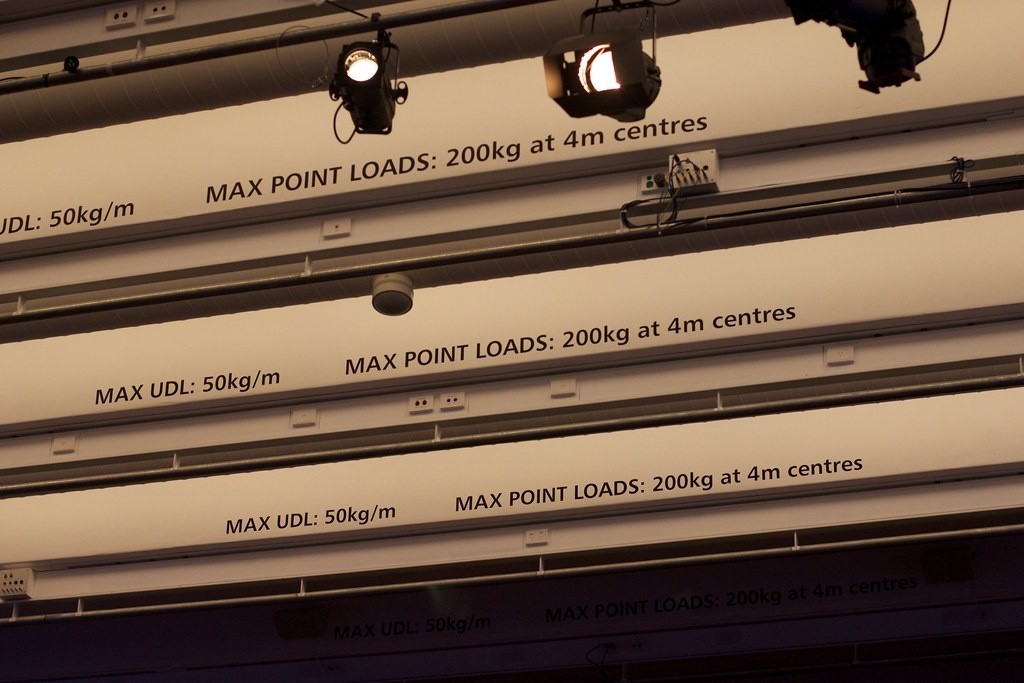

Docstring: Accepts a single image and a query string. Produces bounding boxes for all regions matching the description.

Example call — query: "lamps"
[784,0,925,94]
[543,30,662,122]
[333,39,399,135]
[372,272,414,317]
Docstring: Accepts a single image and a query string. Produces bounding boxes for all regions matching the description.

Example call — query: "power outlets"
[322,216,351,239]
[438,391,465,409]
[408,395,434,413]
[524,528,548,547]
[292,407,317,429]
[826,345,855,367]
[668,149,721,195]
[104,3,138,29]
[52,434,75,456]
[640,167,668,193]
[550,378,577,398]
[143,0,176,22]
[0,569,34,598]
